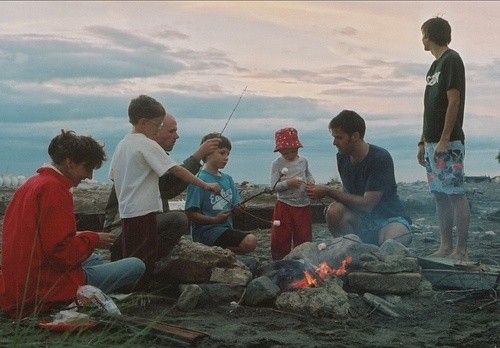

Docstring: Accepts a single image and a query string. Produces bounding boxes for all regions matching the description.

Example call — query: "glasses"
[142,117,164,130]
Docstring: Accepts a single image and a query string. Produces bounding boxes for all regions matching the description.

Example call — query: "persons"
[184,133,258,254]
[110,95,221,294]
[0,129,146,319]
[103,112,223,260]
[305,110,412,248]
[417,17,471,260]
[270,128,316,260]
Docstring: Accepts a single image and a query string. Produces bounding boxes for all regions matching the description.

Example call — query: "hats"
[272,127,304,152]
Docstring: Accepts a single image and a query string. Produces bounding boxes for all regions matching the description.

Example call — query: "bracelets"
[418,139,424,145]
